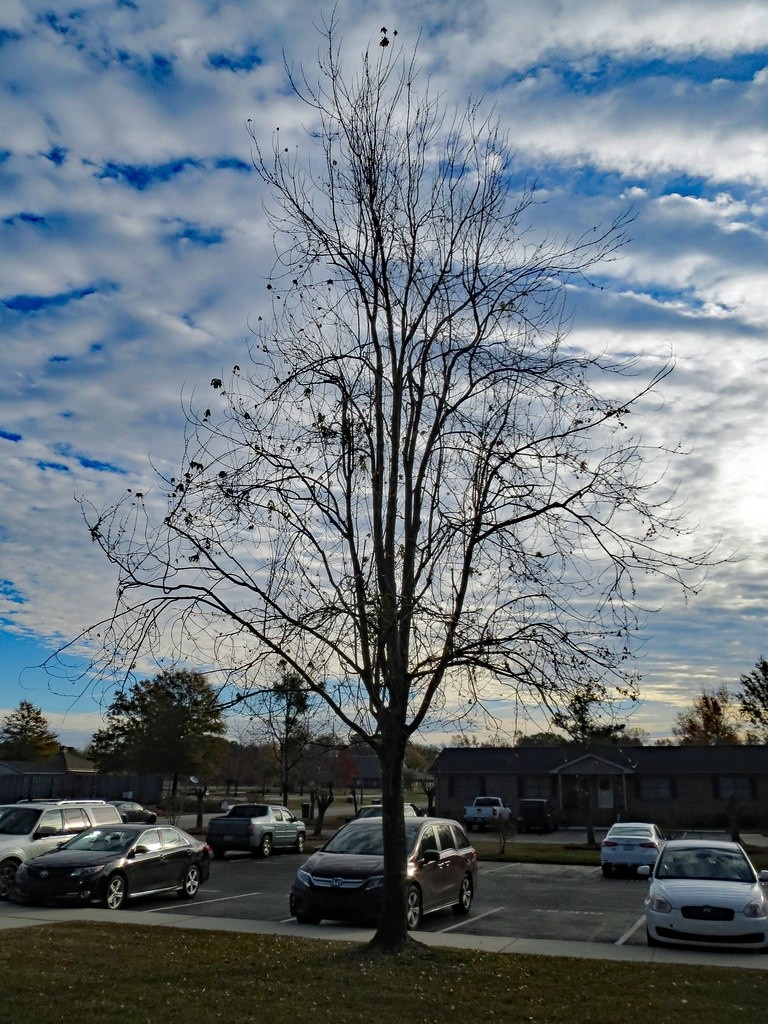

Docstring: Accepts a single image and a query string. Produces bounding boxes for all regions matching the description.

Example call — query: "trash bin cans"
[301,803,311,819]
[372,799,381,805]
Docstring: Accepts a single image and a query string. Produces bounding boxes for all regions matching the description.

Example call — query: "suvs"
[0,799,124,903]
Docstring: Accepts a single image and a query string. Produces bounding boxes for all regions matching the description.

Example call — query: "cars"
[516,799,559,834]
[290,816,479,931]
[354,802,426,818]
[14,824,211,910]
[106,801,157,824]
[636,839,768,956]
[601,823,674,877]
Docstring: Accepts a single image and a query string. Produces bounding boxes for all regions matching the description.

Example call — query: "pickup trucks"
[464,797,512,833]
[206,802,306,859]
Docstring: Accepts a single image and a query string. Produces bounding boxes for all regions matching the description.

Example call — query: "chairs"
[109,835,120,851]
